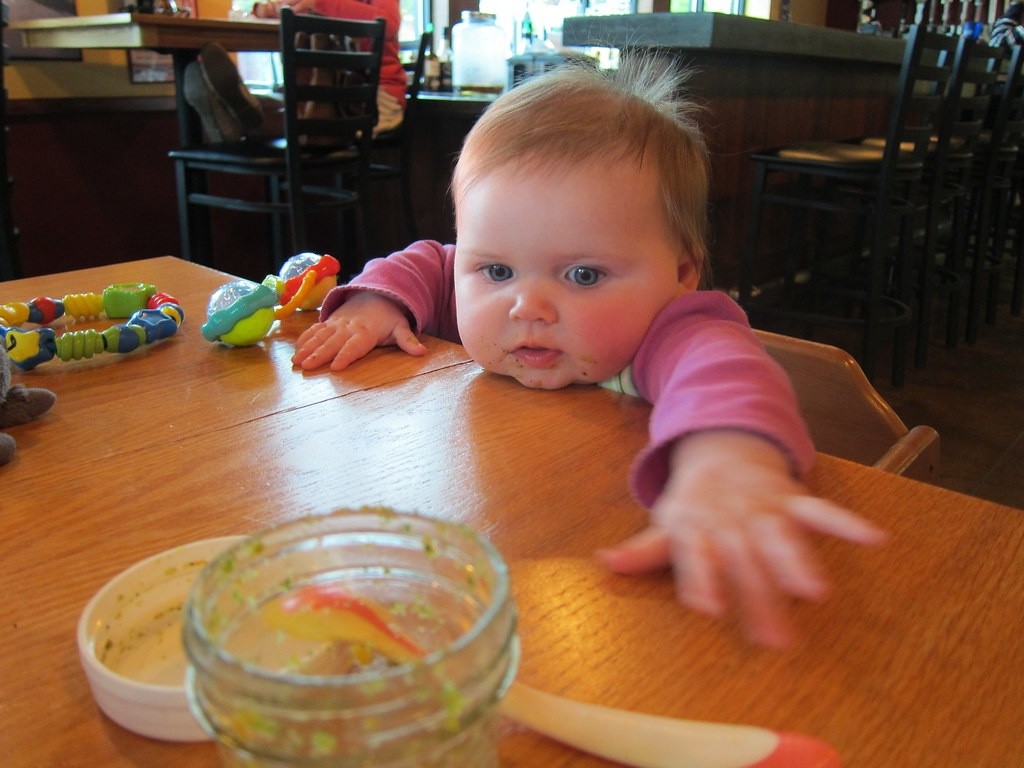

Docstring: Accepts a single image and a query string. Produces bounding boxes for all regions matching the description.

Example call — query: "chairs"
[739,24,1024,387]
[256,31,432,274]
[170,7,388,279]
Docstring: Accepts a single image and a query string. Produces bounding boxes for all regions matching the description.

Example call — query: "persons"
[988,1,1024,48]
[290,69,886,647]
[183,0,408,146]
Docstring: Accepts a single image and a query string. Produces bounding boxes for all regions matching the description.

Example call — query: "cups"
[184,502,524,767]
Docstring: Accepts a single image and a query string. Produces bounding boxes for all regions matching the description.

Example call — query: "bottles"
[424,25,453,92]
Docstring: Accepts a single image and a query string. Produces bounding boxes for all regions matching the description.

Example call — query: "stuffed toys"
[0,346,56,466]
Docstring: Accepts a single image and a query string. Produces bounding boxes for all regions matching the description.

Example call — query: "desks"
[0,255,1024,768]
[11,12,282,270]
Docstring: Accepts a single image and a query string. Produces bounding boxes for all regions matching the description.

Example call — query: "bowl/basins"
[75,533,256,741]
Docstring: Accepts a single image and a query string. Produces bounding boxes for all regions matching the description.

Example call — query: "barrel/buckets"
[450,9,510,91]
